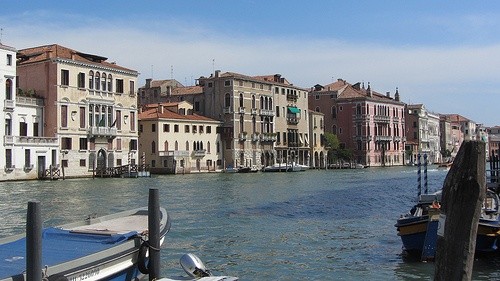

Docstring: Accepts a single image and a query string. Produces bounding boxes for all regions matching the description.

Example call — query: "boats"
[0,205,171,278]
[437,160,454,169]
[395,187,500,257]
[225,166,258,173]
[330,161,365,169]
[154,253,239,281]
[265,163,309,172]
